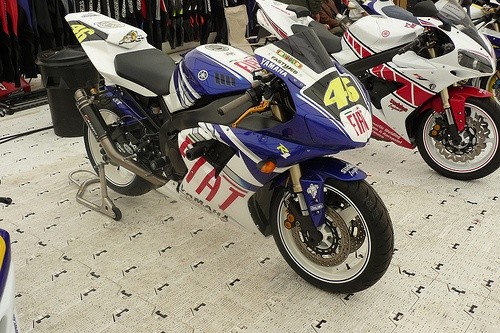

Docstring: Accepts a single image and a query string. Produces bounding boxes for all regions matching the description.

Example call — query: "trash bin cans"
[35,44,100,137]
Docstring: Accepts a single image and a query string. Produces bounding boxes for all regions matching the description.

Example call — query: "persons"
[280,0,365,35]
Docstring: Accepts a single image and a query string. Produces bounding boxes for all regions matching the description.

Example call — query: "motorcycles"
[64,11,394,296]
[252,0,500,181]
